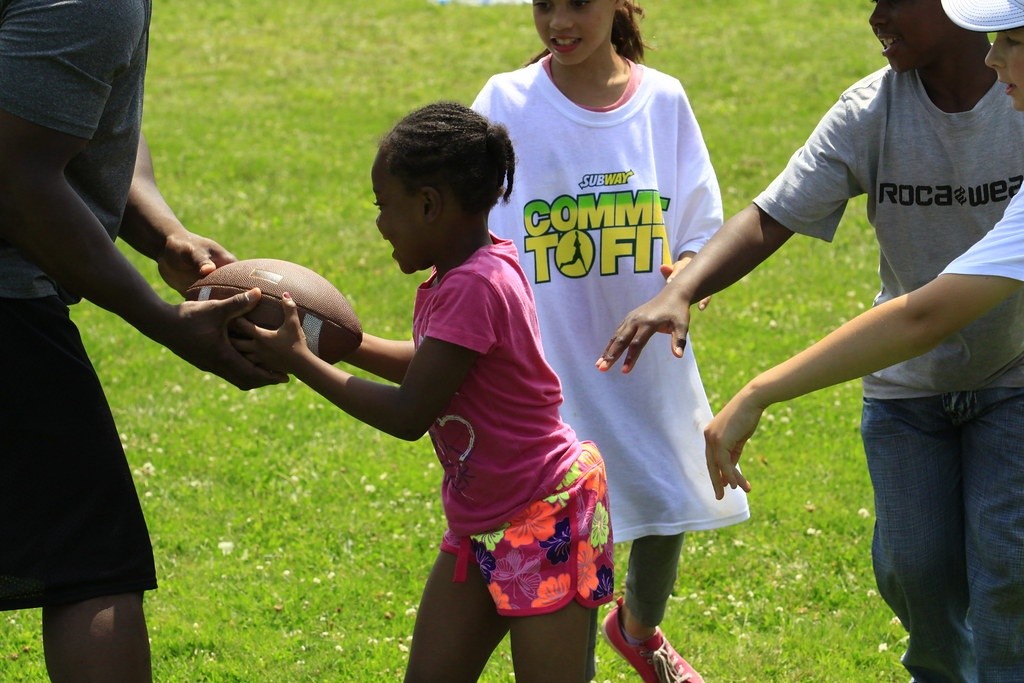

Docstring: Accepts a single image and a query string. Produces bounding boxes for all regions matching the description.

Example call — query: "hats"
[942,0,1023,32]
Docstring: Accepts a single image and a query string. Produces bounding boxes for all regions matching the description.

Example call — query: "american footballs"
[184,258,362,370]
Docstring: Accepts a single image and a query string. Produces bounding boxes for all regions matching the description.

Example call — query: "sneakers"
[603,602,706,683]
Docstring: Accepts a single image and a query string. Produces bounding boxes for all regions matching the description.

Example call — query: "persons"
[597,4,1023,681]
[464,0,752,683]
[703,1,1020,501]
[229,103,615,683]
[0,0,288,681]
